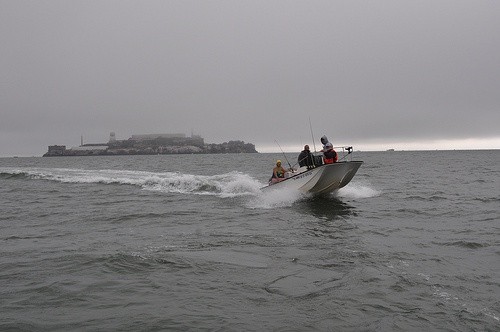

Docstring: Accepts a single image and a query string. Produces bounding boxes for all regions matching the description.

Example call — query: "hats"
[276,159,281,165]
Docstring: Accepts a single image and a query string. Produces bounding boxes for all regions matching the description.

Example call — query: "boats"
[260,146,364,202]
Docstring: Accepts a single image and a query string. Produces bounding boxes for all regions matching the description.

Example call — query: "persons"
[321,136,333,159]
[268,160,286,181]
[298,145,316,168]
[322,144,338,164]
